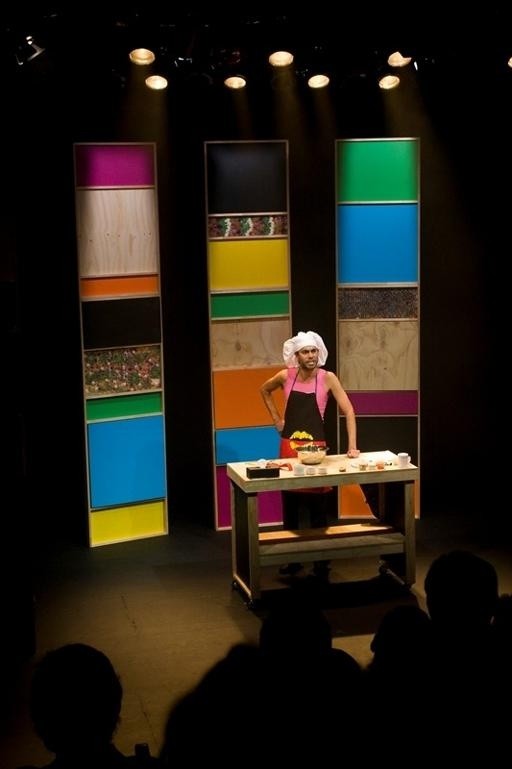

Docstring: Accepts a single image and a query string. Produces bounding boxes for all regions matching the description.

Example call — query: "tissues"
[246,458,280,480]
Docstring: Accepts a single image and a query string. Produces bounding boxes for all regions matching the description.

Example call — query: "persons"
[23,643,138,769]
[258,330,361,581]
[161,551,512,769]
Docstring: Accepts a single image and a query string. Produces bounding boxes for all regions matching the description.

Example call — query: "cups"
[397,452,412,468]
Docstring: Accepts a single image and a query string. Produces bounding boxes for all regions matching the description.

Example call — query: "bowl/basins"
[295,445,329,465]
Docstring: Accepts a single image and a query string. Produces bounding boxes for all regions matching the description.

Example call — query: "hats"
[284,331,329,369]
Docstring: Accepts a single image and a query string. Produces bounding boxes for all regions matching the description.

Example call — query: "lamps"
[12,33,49,66]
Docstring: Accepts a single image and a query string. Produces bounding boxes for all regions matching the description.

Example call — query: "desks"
[226,449,417,611]
